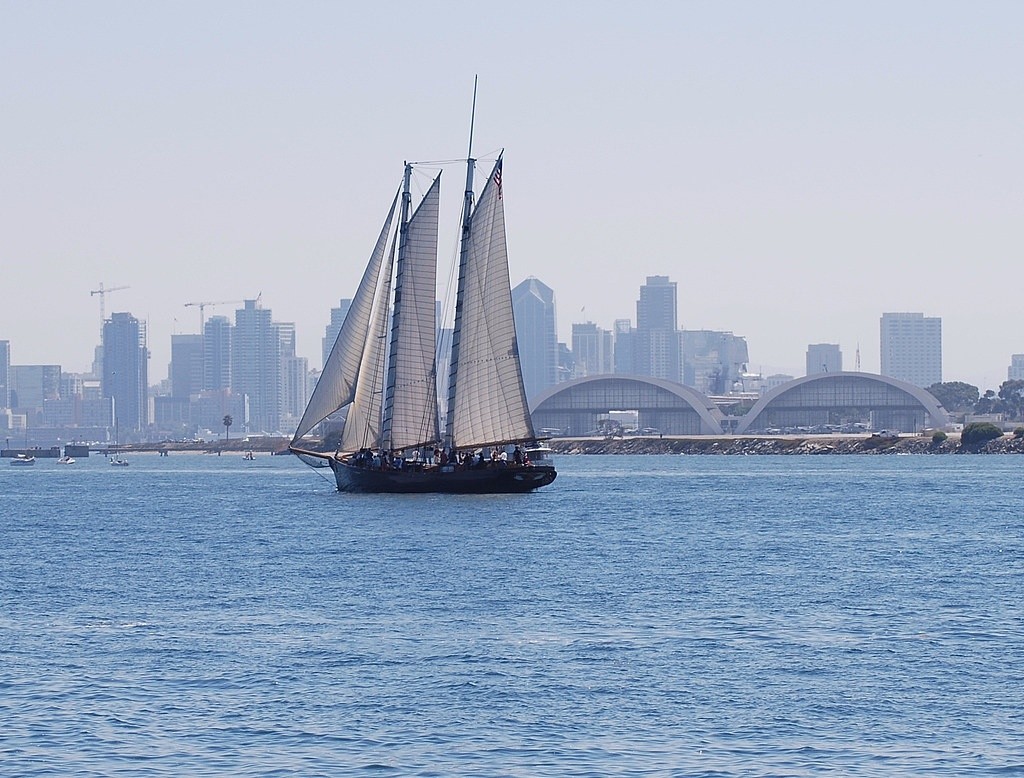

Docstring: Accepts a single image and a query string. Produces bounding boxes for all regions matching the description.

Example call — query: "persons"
[412,448,420,465]
[513,446,530,467]
[434,447,508,464]
[348,447,407,471]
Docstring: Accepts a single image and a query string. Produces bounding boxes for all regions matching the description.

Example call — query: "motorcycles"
[242,454,256,461]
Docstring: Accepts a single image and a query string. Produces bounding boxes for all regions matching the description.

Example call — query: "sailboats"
[287,74,557,493]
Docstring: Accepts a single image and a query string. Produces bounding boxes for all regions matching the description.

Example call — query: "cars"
[872,430,899,437]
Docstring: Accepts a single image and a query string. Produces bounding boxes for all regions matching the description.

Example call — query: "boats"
[110,457,128,466]
[57,456,75,465]
[9,456,36,466]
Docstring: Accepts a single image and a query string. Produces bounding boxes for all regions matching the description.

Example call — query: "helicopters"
[538,427,570,438]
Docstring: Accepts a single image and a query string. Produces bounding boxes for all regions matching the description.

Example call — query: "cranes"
[184,301,240,334]
[90,282,131,344]
